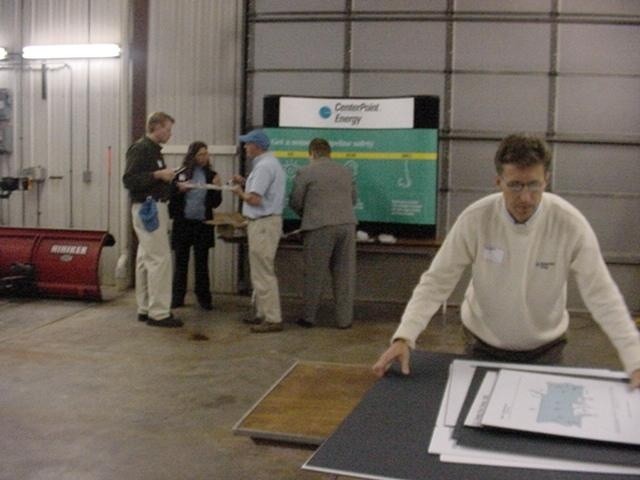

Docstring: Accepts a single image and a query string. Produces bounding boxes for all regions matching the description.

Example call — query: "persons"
[290,138,358,328]
[368,127,640,391]
[168,142,222,310]
[228,128,287,332]
[121,110,186,328]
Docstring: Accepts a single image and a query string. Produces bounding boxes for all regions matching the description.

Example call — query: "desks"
[213,235,446,308]
[231,358,384,451]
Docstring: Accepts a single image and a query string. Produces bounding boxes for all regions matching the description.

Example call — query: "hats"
[237,129,270,149]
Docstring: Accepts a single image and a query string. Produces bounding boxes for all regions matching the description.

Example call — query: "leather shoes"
[244,315,352,333]
[139,301,213,327]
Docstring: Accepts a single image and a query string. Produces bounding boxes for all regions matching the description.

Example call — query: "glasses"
[499,174,547,192]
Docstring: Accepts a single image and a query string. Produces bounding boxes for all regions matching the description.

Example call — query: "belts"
[463,325,567,361]
[132,199,166,202]
